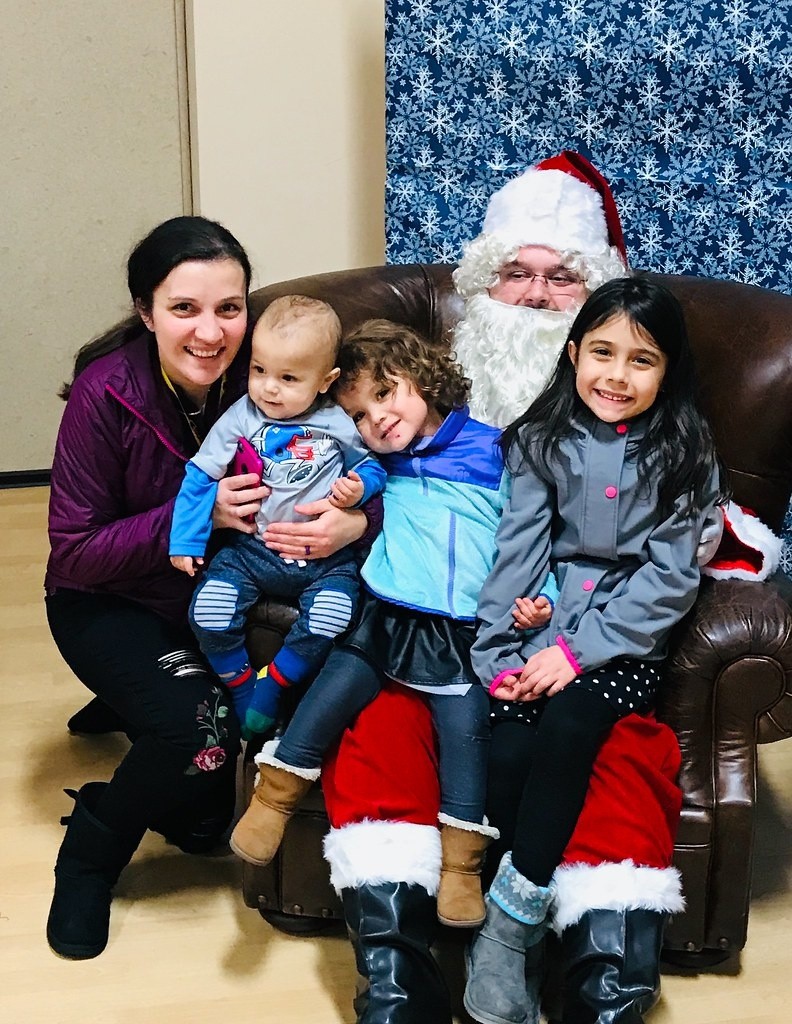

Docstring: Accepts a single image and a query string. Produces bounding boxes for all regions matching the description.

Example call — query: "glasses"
[496,265,589,287]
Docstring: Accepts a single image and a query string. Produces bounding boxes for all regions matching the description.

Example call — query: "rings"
[305,546,311,555]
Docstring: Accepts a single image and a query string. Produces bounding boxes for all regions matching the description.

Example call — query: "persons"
[323,150,783,1024]
[43,216,383,959]
[169,295,388,741]
[463,278,722,1024]
[228,317,509,927]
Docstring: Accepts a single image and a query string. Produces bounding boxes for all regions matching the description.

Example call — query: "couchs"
[206,265,792,981]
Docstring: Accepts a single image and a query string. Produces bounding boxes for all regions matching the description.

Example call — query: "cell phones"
[230,436,266,524]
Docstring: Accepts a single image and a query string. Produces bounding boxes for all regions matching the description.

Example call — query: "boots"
[67,695,143,743]
[434,812,501,928]
[44,781,146,963]
[452,850,556,1024]
[542,858,688,1024]
[319,817,454,1024]
[229,740,322,866]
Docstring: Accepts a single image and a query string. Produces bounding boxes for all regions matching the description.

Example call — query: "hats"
[449,150,630,431]
